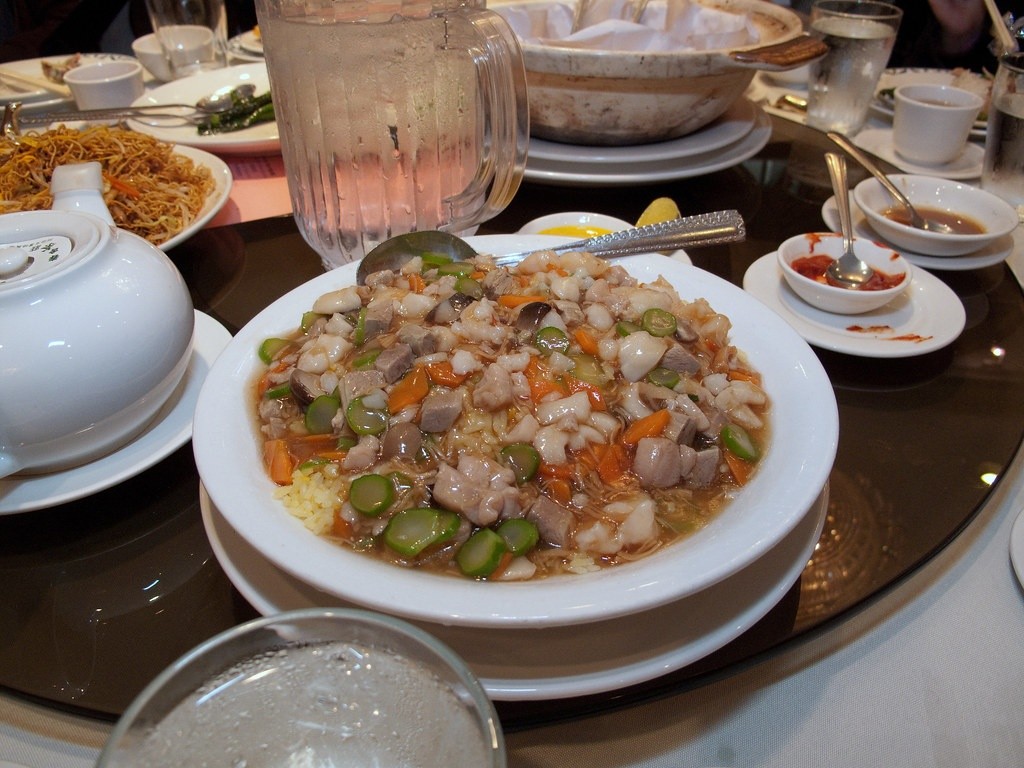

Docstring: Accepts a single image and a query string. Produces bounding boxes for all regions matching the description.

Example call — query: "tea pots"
[0,160,195,484]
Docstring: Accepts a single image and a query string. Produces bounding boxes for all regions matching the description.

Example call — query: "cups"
[93,606,507,768]
[983,54,1024,223]
[805,0,903,139]
[145,0,230,79]
[891,83,985,167]
[252,0,531,273]
[63,61,145,111]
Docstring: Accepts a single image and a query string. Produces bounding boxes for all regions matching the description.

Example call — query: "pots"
[484,0,831,147]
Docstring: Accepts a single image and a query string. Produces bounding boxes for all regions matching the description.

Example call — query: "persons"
[0,0,128,63]
[885,0,1024,75]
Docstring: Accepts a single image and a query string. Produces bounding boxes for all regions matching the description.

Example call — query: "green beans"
[197,87,277,135]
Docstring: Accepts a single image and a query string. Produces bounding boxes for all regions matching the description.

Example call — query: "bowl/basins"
[776,231,914,316]
[191,232,840,628]
[0,54,140,118]
[853,172,1020,257]
[131,25,215,84]
[515,211,693,266]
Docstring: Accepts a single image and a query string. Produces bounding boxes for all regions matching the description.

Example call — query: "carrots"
[263,273,749,575]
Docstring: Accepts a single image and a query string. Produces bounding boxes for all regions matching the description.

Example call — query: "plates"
[226,31,266,62]
[156,144,233,253]
[742,250,966,358]
[0,310,236,515]
[196,478,829,703]
[485,97,774,186]
[821,189,1015,270]
[869,67,987,139]
[127,62,282,159]
[855,128,985,179]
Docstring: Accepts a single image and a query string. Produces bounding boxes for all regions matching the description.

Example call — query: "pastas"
[0,122,214,246]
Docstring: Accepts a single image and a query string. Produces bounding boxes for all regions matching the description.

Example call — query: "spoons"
[354,208,746,288]
[823,152,873,291]
[17,84,256,124]
[828,130,956,236]
